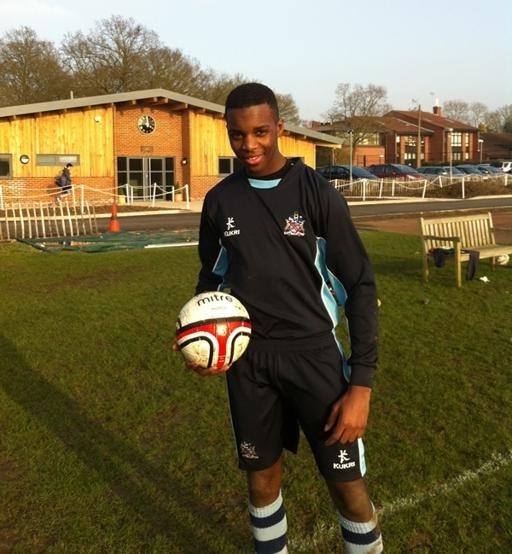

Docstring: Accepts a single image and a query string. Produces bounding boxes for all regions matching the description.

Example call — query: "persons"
[194,81,381,552]
[53,161,73,193]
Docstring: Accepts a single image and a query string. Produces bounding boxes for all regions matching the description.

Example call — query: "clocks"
[138,115,156,134]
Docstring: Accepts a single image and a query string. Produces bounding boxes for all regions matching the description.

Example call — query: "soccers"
[496,254,510,266]
[175,291,253,369]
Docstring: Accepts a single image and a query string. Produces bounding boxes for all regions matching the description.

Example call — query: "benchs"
[419,212,512,288]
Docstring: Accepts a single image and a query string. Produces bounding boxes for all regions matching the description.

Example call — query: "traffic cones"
[108,202,122,234]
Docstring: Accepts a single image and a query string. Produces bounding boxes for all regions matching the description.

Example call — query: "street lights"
[348,129,354,190]
[445,127,453,182]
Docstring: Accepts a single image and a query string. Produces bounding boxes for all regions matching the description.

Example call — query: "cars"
[317,161,512,180]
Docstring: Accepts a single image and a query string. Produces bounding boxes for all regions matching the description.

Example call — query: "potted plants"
[174,181,183,202]
[118,186,126,204]
